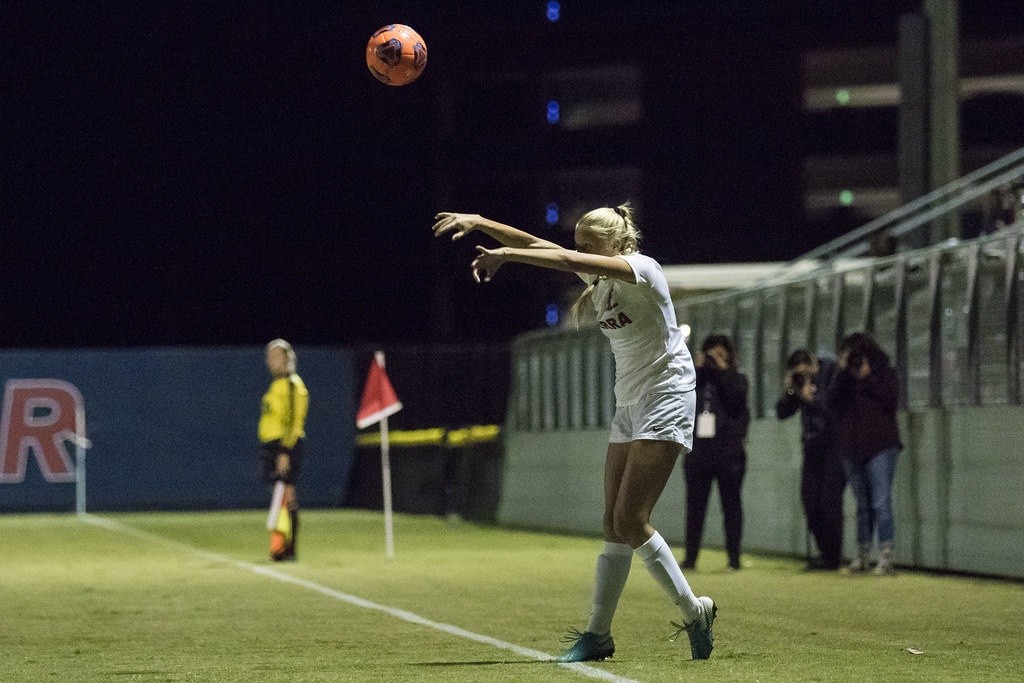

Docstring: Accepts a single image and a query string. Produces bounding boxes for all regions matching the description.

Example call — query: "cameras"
[792,372,805,388]
[703,356,715,368]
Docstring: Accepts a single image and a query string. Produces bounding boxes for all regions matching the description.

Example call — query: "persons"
[678,333,750,572]
[822,331,907,576]
[776,347,849,573]
[258,338,309,563]
[432,208,718,662]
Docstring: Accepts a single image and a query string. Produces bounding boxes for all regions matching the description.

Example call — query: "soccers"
[365,23,428,87]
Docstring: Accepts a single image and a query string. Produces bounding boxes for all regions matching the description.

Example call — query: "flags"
[356,357,403,430]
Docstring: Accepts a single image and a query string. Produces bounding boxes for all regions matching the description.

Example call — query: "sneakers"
[840,560,872,573]
[553,626,615,664]
[876,560,895,576]
[669,595,719,660]
